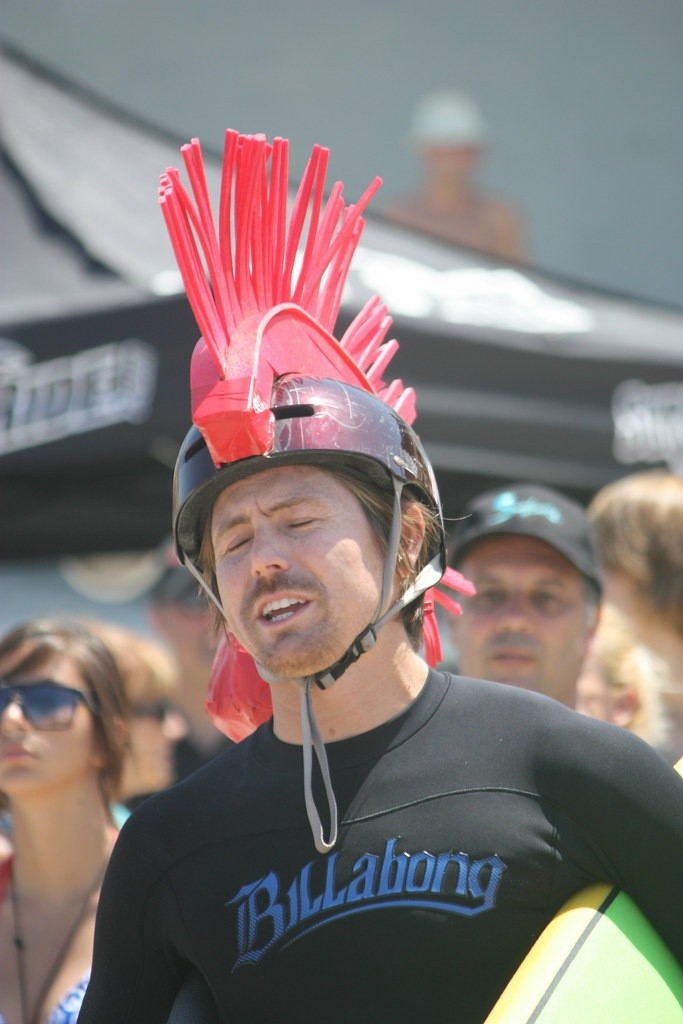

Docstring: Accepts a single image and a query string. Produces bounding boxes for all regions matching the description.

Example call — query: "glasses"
[0,684,98,732]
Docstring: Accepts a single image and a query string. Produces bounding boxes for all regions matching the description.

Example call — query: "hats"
[444,483,599,583]
[147,538,212,604]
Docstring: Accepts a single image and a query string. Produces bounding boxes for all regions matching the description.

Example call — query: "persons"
[445,467,683,781]
[75,376,683,1024]
[0,560,248,1024]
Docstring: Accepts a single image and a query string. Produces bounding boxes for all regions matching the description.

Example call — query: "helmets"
[156,130,442,568]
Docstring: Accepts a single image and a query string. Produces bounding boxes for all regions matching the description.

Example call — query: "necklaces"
[7,856,107,1024]
